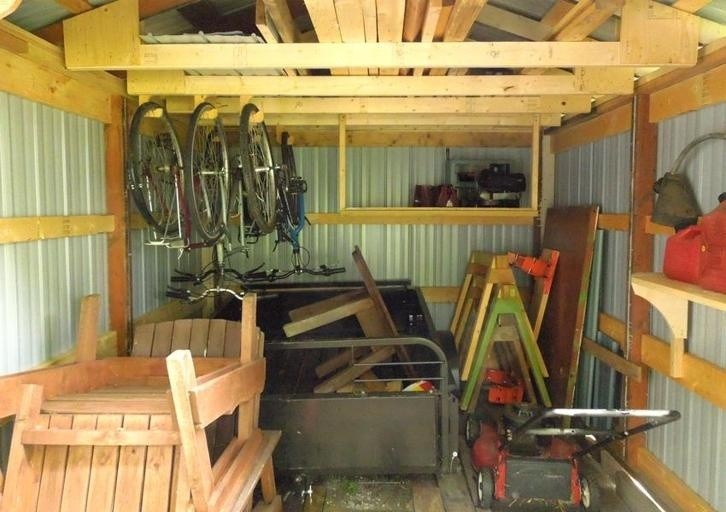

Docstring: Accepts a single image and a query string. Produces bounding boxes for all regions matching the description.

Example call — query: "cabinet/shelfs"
[127,114,540,222]
[630,270,726,339]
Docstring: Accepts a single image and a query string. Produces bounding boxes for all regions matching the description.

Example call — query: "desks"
[281,244,415,395]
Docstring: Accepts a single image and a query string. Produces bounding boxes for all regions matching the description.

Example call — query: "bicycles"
[170,103,265,285]
[126,99,278,306]
[236,103,347,281]
